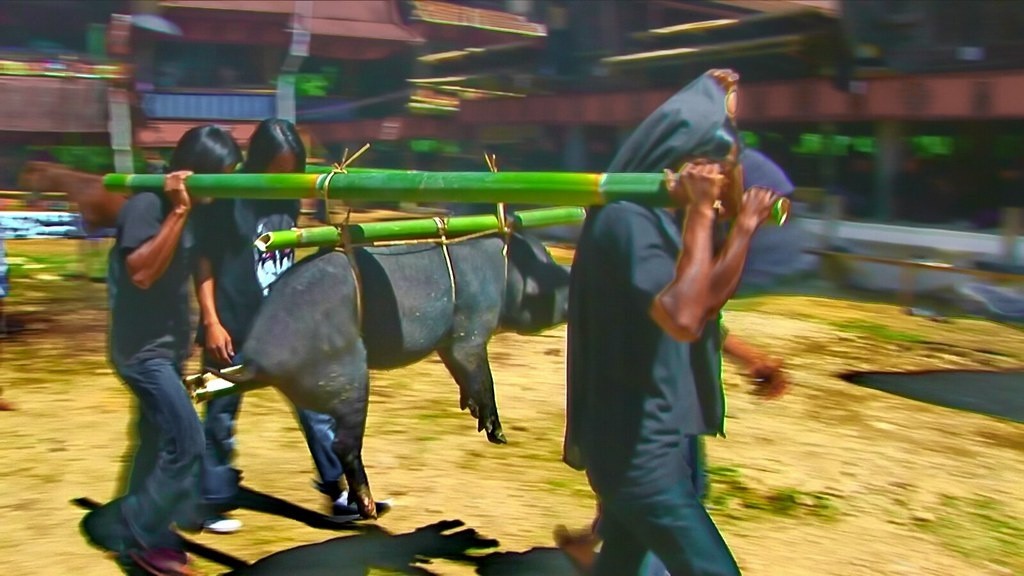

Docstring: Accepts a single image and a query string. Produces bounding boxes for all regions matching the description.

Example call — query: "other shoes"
[330,493,391,524]
[196,511,244,533]
[82,504,190,576]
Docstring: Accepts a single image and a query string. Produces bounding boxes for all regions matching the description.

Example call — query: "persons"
[552,68,795,576]
[78,117,391,576]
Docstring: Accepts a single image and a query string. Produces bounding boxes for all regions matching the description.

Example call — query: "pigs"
[202,209,570,521]
